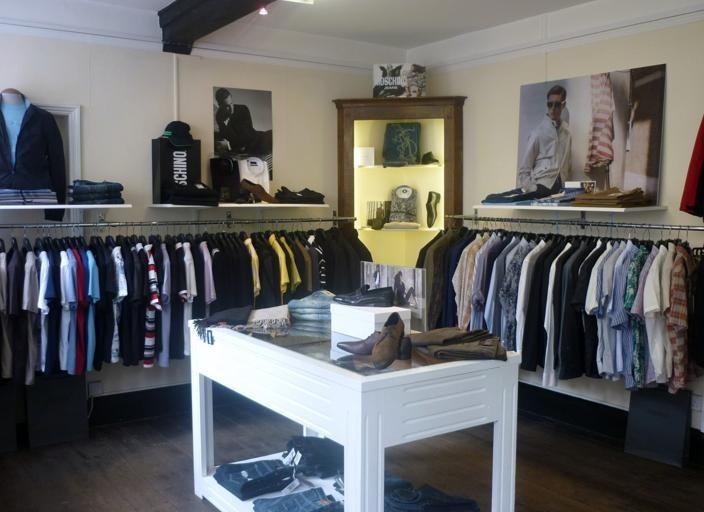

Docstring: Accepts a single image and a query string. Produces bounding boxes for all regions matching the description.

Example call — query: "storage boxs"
[329,302,412,340]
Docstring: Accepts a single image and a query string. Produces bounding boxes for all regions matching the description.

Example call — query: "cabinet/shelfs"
[332,97,464,269]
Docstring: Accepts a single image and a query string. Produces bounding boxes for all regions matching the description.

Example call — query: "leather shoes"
[241,179,279,203]
[372,312,404,370]
[426,192,440,228]
[337,330,412,360]
[372,218,383,229]
[334,284,395,307]
[422,152,438,164]
[368,217,388,226]
[236,192,262,203]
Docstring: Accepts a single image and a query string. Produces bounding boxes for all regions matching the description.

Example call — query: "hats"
[163,121,194,147]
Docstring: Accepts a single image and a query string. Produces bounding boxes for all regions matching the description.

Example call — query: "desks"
[187,317,523,512]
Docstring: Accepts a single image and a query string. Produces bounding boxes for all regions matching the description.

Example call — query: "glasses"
[547,100,565,108]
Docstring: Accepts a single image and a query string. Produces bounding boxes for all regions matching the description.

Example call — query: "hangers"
[0,215,356,256]
[442,211,691,250]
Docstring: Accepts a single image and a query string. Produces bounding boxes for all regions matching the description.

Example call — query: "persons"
[0,87,67,223]
[394,271,419,307]
[373,264,380,288]
[520,84,573,194]
[215,88,262,155]
